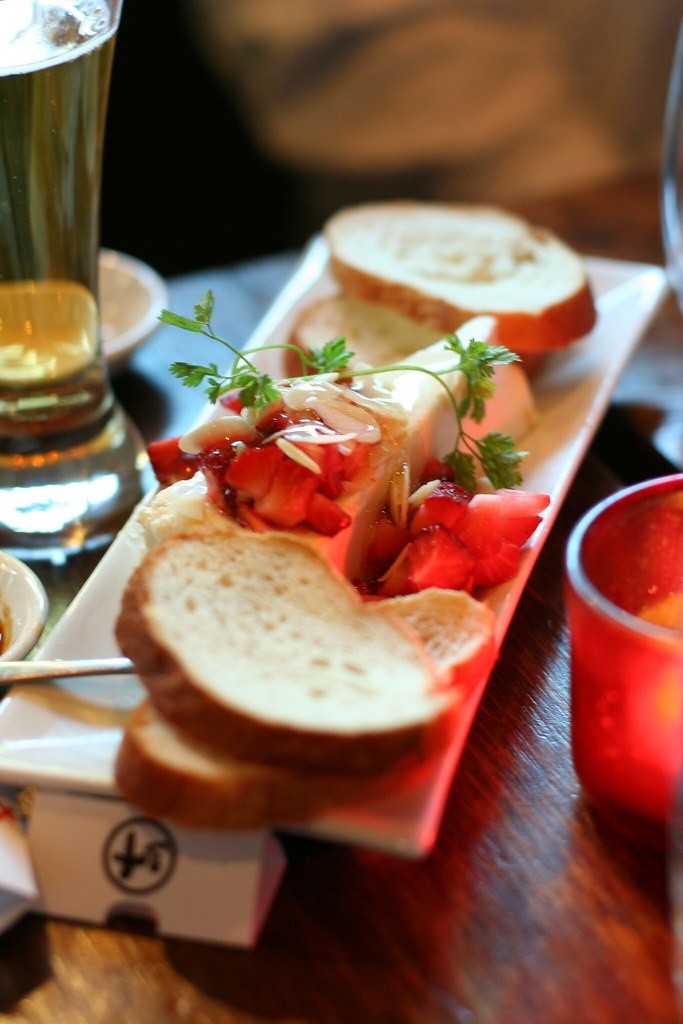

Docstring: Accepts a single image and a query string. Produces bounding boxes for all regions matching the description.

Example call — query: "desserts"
[137,314,537,585]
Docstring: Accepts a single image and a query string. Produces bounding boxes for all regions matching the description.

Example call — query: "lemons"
[0,281,101,381]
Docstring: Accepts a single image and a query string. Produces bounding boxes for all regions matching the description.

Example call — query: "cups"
[563,472,683,868]
[1,0,158,564]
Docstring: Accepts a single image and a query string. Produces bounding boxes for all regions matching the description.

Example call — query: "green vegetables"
[155,287,529,496]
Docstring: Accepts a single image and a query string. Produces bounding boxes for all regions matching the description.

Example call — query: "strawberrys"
[367,458,552,600]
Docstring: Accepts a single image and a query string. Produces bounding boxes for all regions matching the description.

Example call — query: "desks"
[0,174,683,1024]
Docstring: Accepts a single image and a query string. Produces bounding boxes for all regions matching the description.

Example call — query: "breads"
[285,292,437,378]
[321,197,597,354]
[116,533,497,827]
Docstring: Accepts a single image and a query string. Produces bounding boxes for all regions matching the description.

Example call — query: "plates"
[0,790,38,939]
[92,244,165,371]
[0,218,670,856]
[1,549,51,669]
